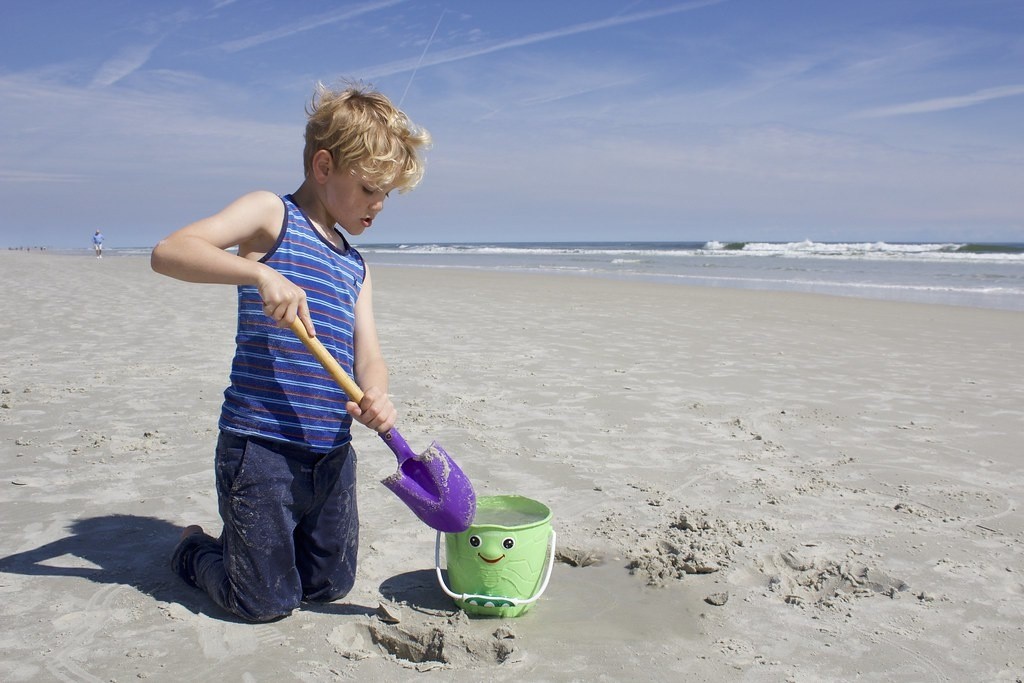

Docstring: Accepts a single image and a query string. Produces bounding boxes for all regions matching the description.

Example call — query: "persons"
[151,75,431,622]
[91,229,104,259]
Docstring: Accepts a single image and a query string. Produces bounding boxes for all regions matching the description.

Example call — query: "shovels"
[284,311,476,534]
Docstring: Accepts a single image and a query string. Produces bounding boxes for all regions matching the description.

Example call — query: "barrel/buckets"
[435,494,556,617]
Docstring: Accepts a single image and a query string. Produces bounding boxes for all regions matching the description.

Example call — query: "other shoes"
[96,256,99,258]
[99,255,102,258]
